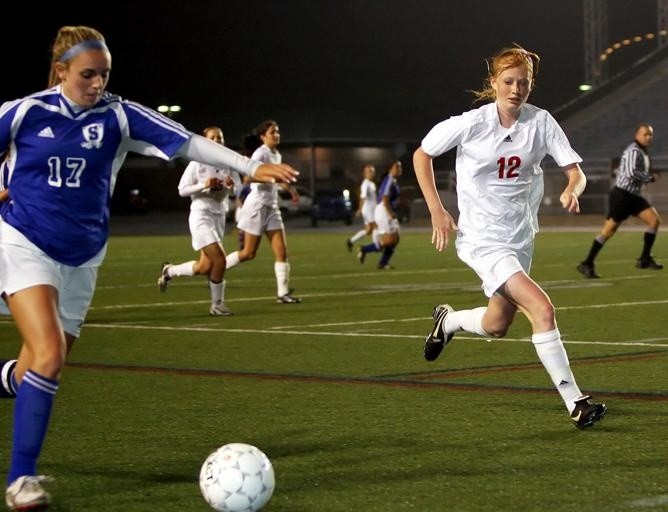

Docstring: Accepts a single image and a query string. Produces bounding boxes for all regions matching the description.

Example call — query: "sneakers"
[375,262,394,271]
[273,291,301,305]
[576,260,601,281]
[156,261,171,291]
[422,302,453,361]
[635,255,664,271]
[207,301,236,319]
[1,473,54,509]
[357,243,366,263]
[346,238,354,252]
[565,393,607,428]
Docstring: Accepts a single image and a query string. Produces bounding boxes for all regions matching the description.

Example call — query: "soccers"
[199,442,275,512]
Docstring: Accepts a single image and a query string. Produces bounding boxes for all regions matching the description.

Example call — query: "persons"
[156,124,242,316]
[358,158,404,271]
[347,163,380,252]
[210,119,300,306]
[577,122,663,278]
[412,44,607,430]
[1,26,300,512]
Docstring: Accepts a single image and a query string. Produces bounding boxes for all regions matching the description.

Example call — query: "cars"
[272,186,313,220]
[310,185,355,226]
